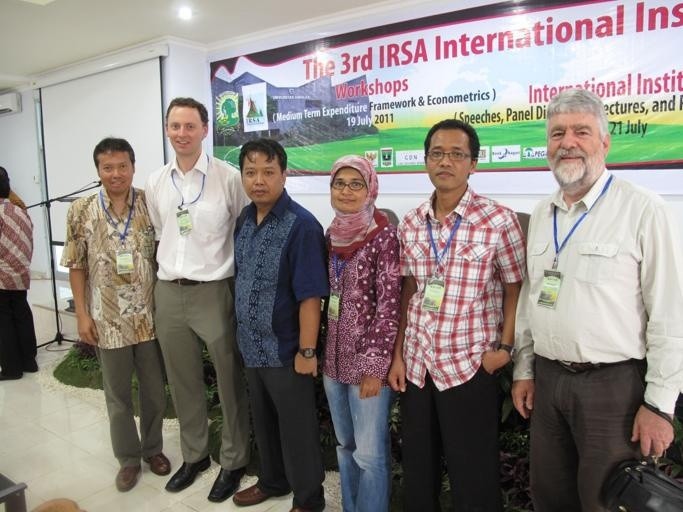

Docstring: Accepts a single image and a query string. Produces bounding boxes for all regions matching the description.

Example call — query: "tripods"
[31,202,82,350]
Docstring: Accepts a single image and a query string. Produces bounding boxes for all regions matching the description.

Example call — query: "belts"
[551,359,587,376]
[172,278,202,287]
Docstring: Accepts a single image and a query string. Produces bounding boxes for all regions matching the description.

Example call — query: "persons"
[29,496,83,512]
[388,118,526,512]
[232,138,326,512]
[144,96,254,503]
[60,137,172,494]
[510,88,682,512]
[0,166,39,382]
[320,153,400,512]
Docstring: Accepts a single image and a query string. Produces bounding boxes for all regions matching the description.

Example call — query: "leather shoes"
[116,462,141,492]
[165,455,211,491]
[233,484,268,506]
[145,453,170,475]
[208,465,246,502]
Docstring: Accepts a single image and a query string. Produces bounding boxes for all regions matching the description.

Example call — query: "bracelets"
[498,341,511,354]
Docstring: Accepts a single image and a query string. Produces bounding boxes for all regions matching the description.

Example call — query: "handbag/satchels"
[599,454,682,512]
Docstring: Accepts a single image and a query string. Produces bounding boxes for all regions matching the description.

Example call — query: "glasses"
[429,149,468,162]
[332,181,366,191]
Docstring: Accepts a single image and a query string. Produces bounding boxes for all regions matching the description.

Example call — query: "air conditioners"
[0,92,21,116]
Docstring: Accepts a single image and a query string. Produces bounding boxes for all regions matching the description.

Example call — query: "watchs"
[296,347,316,359]
